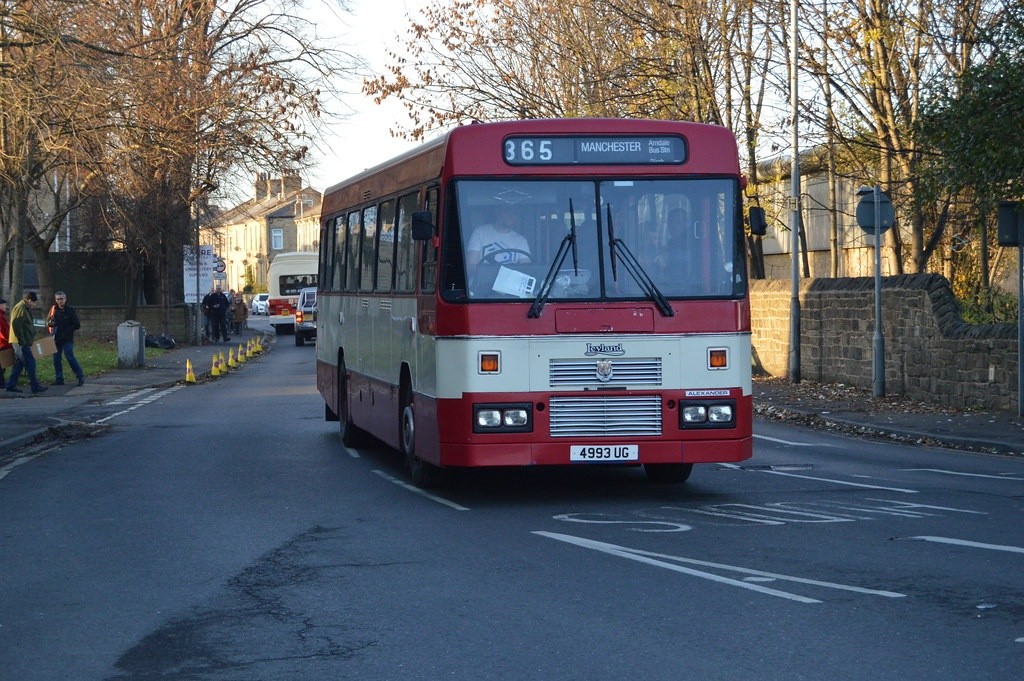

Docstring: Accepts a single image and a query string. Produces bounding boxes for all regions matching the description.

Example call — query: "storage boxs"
[0,348,17,369]
[31,336,58,358]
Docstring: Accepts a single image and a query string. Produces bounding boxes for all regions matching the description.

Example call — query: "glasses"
[57,297,65,300]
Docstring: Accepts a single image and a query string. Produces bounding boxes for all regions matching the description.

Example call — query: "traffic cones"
[251,339,257,352]
[237,343,246,362]
[219,352,227,372]
[227,347,237,368]
[246,340,253,357]
[211,355,221,377]
[256,336,263,351]
[186,358,197,384]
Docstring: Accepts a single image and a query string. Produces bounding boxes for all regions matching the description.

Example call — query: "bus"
[268,252,320,336]
[317,118,768,491]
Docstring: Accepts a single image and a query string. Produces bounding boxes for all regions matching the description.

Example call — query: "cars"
[291,287,318,347]
[264,297,269,315]
[252,294,269,316]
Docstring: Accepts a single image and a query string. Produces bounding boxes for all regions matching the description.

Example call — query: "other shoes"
[50,382,64,385]
[6,386,22,393]
[32,385,48,394]
[78,377,84,386]
[223,337,231,342]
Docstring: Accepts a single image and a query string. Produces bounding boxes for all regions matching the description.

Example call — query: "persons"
[657,209,700,292]
[228,293,248,336]
[227,289,236,332]
[632,220,667,292]
[467,202,532,292]
[300,277,308,287]
[5,292,48,393]
[46,292,85,386]
[206,284,231,342]
[202,287,214,341]
[0,299,10,389]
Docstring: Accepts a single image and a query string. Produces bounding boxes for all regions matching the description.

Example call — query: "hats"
[0,298,7,304]
[24,292,37,301]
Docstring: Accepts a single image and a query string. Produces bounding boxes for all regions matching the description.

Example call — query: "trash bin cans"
[117,320,145,368]
